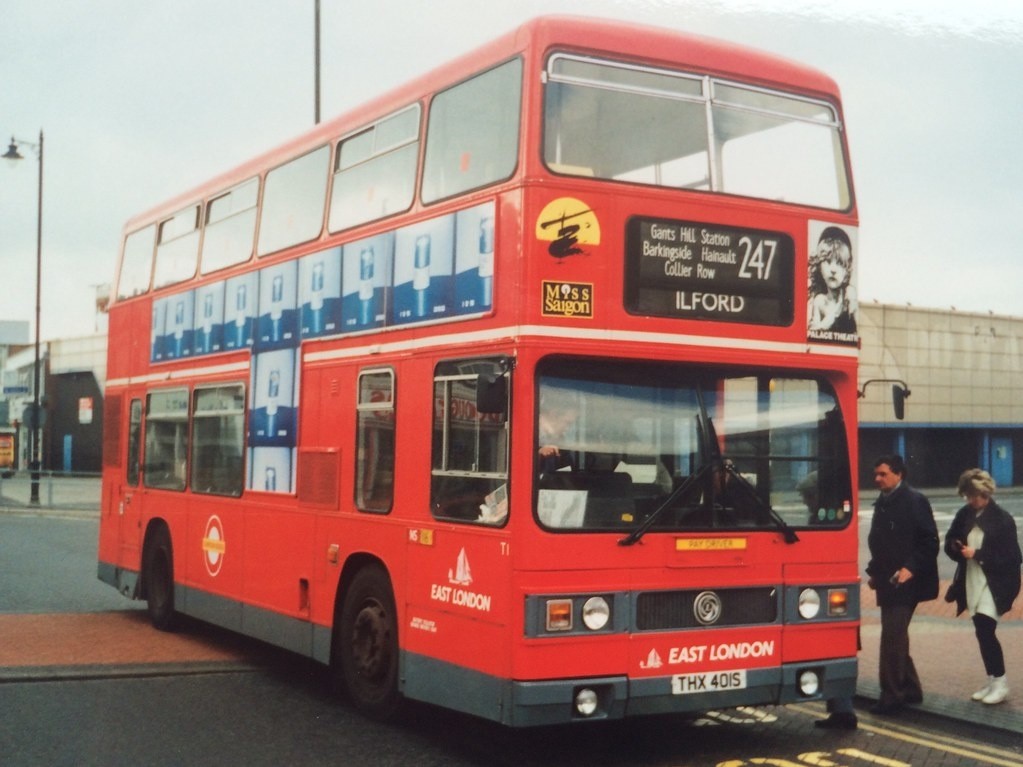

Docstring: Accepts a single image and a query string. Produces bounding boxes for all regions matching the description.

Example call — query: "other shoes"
[868,703,905,715]
[906,694,924,704]
[814,713,858,730]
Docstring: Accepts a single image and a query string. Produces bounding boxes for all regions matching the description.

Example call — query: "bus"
[96,13,911,733]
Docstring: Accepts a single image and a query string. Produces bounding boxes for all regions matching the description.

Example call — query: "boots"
[972,674,996,700]
[982,672,1011,705]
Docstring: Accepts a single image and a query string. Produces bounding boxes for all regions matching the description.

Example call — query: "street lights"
[0,128,44,509]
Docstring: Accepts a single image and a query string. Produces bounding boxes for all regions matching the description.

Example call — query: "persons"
[795,471,864,730]
[490,399,582,492]
[690,457,773,528]
[943,468,1023,705]
[865,455,941,714]
[537,393,673,496]
